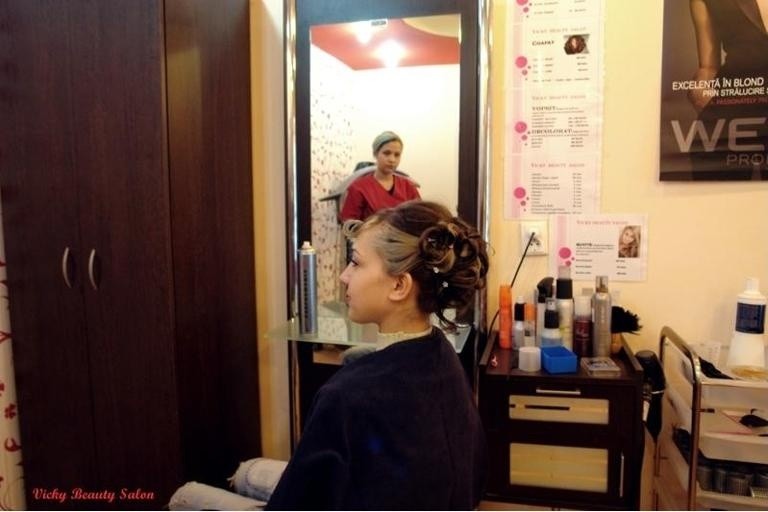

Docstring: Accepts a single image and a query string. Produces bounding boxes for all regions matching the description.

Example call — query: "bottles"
[590,274,611,362]
[297,241,319,335]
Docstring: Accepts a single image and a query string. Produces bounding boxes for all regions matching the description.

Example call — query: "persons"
[339,131,422,228]
[618,226,642,259]
[688,0,768,181]
[170,201,489,510]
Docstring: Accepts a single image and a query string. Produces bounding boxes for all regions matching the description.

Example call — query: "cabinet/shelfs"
[479,327,644,510]
[0,0,264,510]
[654,327,768,511]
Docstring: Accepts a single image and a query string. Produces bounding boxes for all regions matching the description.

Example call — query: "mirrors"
[290,1,479,347]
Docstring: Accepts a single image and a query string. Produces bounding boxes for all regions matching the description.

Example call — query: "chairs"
[320,166,411,266]
